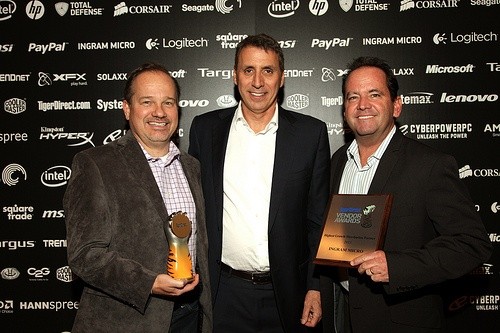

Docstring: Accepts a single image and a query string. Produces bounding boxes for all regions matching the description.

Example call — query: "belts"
[219,260,271,284]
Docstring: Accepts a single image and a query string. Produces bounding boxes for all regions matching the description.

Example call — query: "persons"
[186,32,334,332]
[319,56,491,333]
[62,63,213,332]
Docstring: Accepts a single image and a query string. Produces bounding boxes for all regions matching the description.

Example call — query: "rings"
[370,267,375,275]
